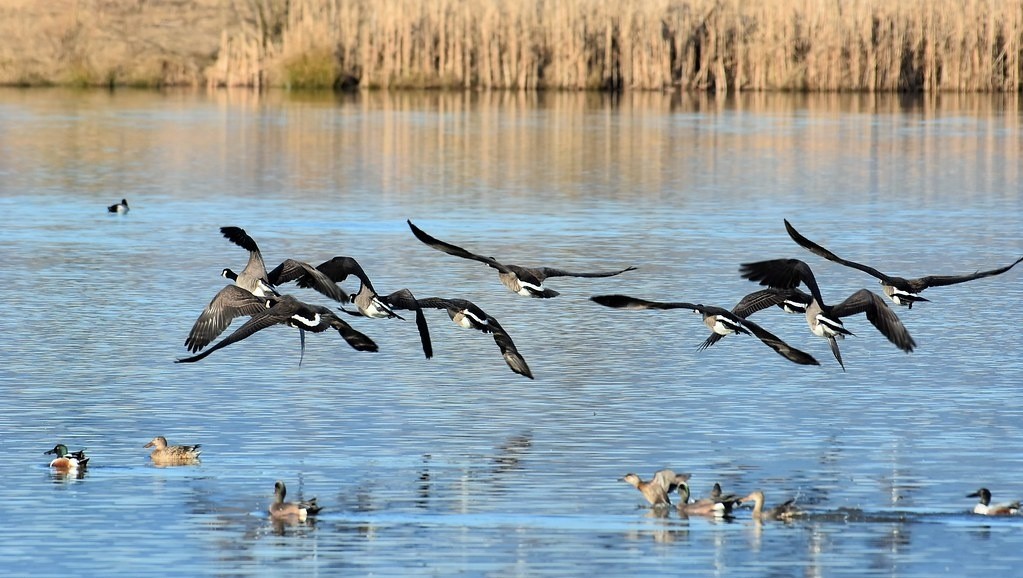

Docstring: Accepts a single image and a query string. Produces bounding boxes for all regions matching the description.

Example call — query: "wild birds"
[693,286,845,354]
[616,469,693,507]
[219,226,352,308]
[675,480,807,521]
[143,436,203,462]
[965,488,1023,516]
[107,199,130,214]
[184,284,305,371]
[172,294,379,364]
[44,443,90,468]
[783,218,1021,310]
[408,297,535,380]
[407,219,639,299]
[268,480,324,517]
[739,258,917,373]
[587,294,821,367]
[294,256,434,360]
